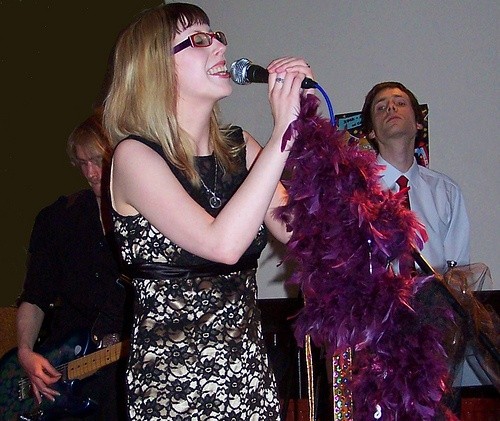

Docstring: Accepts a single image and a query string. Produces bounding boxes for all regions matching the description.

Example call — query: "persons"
[360,81,467,420]
[14,109,138,420]
[99,2,317,421]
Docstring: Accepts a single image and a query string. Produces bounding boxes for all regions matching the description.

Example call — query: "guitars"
[0,334,129,421]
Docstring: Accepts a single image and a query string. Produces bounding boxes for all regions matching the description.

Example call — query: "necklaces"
[192,152,222,209]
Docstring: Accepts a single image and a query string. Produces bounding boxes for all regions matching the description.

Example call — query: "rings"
[305,63,310,68]
[276,77,285,84]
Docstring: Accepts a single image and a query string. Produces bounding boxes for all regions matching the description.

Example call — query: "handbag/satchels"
[90,272,139,348]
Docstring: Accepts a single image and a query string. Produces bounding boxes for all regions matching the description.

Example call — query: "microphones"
[230,58,318,89]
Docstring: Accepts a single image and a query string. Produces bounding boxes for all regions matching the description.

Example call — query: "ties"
[396,175,416,278]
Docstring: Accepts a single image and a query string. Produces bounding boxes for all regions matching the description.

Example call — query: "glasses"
[173,31,227,54]
